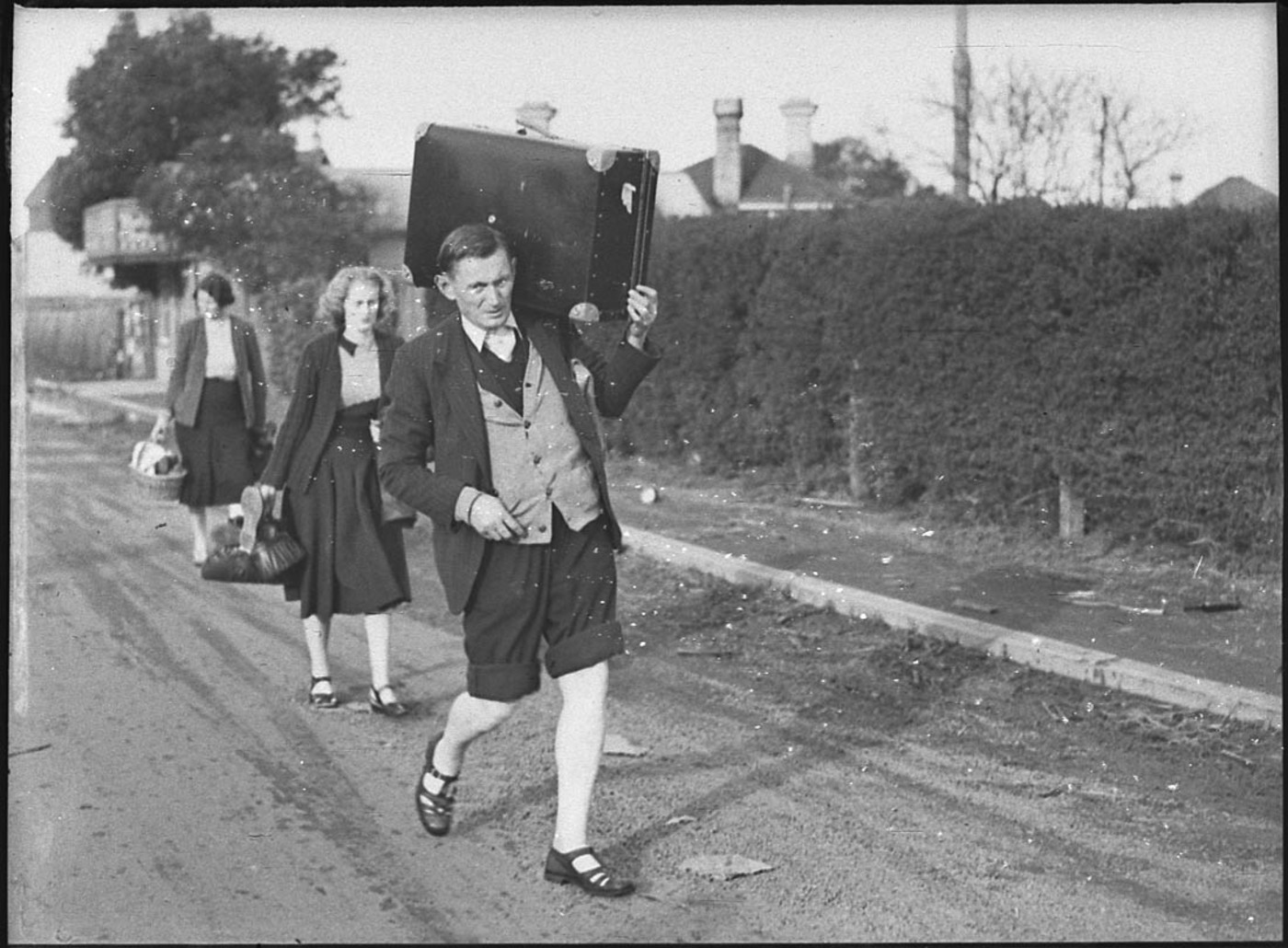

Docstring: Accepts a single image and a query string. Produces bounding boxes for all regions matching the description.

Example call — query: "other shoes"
[239,486,263,551]
[195,554,207,566]
[229,516,243,528]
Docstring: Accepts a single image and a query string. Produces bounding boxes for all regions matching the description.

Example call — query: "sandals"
[308,676,338,707]
[370,684,410,718]
[544,847,635,897]
[415,731,460,836]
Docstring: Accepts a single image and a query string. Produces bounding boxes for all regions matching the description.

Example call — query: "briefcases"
[402,117,660,326]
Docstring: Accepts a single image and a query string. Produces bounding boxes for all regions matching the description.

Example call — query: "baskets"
[126,436,189,509]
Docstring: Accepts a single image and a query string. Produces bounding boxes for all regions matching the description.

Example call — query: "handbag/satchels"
[201,483,307,584]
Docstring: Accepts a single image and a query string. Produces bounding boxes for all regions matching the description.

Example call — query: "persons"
[252,267,434,718]
[151,274,266,566]
[378,225,666,895]
